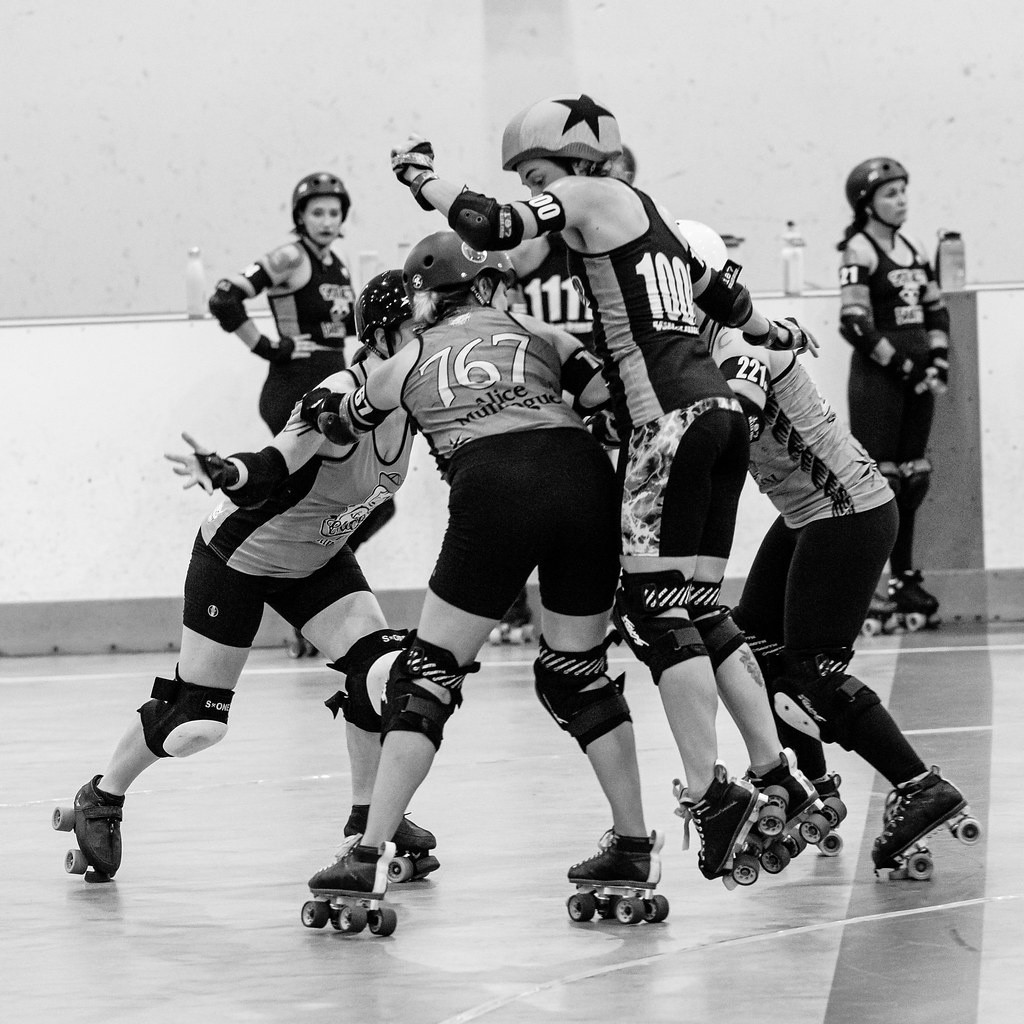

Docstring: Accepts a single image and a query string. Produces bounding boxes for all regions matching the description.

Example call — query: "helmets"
[292,173,350,222]
[502,92,623,172]
[846,158,908,210]
[354,270,411,340]
[403,232,518,306]
[674,220,729,272]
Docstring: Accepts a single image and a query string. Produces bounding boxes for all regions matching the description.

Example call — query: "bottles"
[936,227,966,289]
[779,221,805,294]
[185,247,207,319]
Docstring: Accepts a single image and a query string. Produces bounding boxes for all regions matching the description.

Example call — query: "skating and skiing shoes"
[301,842,397,936]
[567,825,669,925]
[53,775,125,883]
[673,744,847,885]
[345,804,440,882]
[871,766,981,881]
[859,568,940,637]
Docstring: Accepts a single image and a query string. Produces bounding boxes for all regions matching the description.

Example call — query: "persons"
[285,229,665,895]
[389,89,822,880]
[578,219,972,872]
[73,268,438,874]
[205,173,359,440]
[838,156,951,621]
